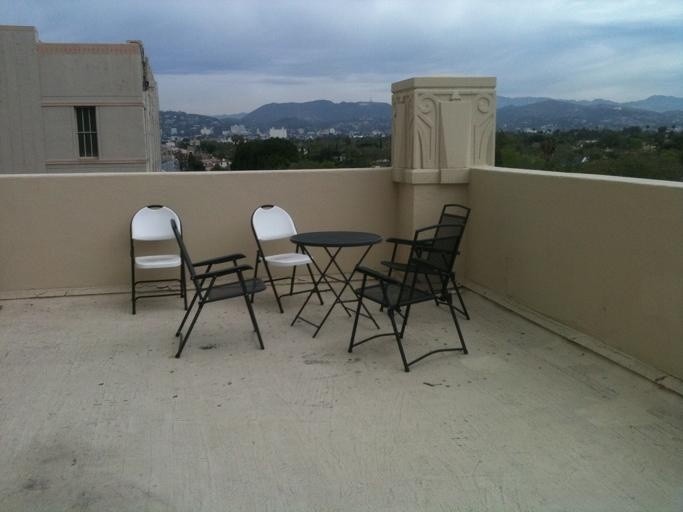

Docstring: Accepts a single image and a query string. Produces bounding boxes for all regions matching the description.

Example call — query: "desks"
[288,231,384,339]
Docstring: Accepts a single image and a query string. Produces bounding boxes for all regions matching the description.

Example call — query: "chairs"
[128,204,188,315]
[168,218,267,358]
[251,204,324,314]
[347,223,469,373]
[379,202,473,322]
